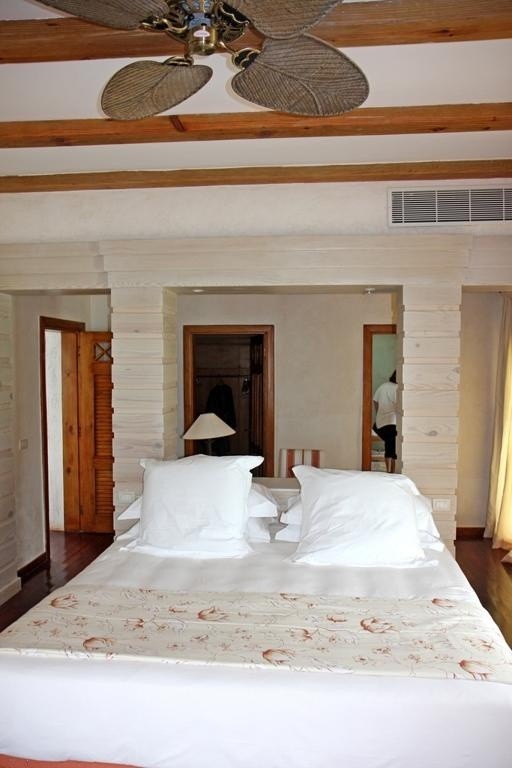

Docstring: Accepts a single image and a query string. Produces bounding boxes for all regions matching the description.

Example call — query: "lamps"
[181,413,236,458]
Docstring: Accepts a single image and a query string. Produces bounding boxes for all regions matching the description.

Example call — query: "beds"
[2,538,512,767]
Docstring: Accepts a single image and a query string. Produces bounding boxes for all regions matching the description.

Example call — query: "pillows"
[277,463,446,568]
[117,454,281,561]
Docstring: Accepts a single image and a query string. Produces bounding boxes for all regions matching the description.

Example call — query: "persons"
[372,370,397,473]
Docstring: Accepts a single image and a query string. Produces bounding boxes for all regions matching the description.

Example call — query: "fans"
[34,0,370,124]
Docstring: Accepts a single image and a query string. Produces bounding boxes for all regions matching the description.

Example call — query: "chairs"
[279,447,326,478]
